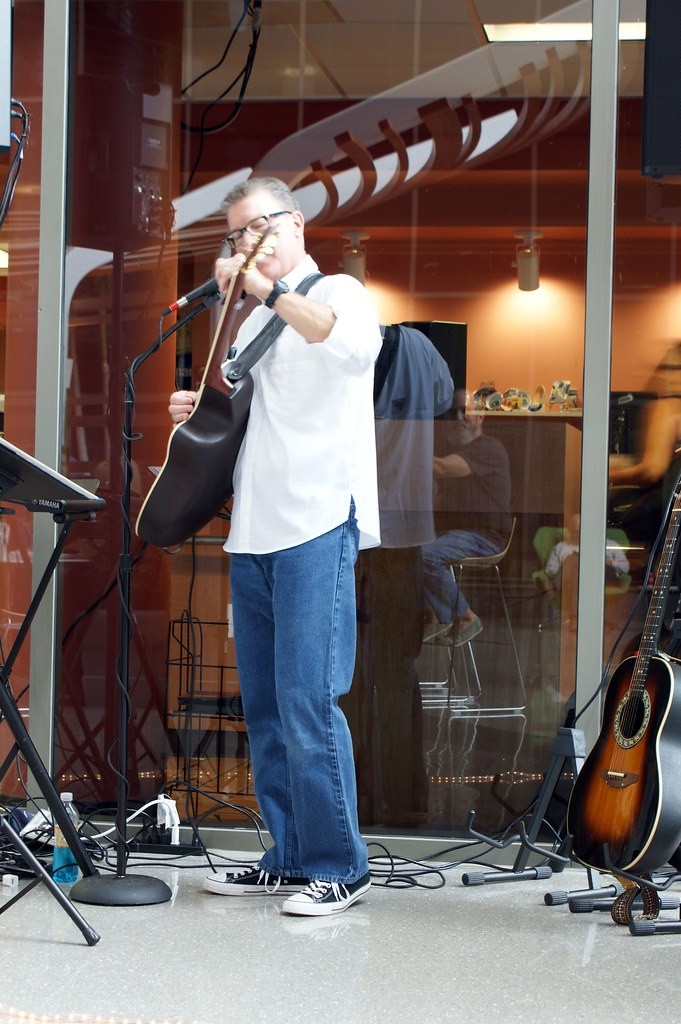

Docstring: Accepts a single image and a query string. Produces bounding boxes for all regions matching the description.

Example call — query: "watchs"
[264,278,290,308]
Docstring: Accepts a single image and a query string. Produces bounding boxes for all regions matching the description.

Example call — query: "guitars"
[563,464,681,888]
[122,212,293,544]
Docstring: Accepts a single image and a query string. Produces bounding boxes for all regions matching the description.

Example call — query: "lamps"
[511,138,545,291]
[340,231,370,287]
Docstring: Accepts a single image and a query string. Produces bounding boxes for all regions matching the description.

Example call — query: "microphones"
[161,278,218,316]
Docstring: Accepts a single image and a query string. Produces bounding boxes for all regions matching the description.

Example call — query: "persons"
[607,335,681,651]
[166,179,381,919]
[338,238,512,840]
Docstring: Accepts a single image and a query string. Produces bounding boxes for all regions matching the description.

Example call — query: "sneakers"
[203,863,311,896]
[283,868,371,916]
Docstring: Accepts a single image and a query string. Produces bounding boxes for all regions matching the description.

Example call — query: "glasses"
[220,210,292,249]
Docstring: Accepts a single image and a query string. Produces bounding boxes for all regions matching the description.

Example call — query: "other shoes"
[529,385,546,412]
[422,619,454,643]
[442,614,482,647]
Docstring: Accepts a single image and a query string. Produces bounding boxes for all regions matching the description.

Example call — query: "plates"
[470,380,547,412]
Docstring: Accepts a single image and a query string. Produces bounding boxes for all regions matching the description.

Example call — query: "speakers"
[400,322,469,421]
[71,78,174,253]
[640,0,681,182]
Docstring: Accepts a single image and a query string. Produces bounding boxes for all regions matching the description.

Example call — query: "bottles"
[52,792,79,884]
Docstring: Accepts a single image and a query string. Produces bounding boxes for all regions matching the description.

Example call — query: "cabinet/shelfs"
[423,408,583,738]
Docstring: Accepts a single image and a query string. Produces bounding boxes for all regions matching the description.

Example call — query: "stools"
[422,515,528,714]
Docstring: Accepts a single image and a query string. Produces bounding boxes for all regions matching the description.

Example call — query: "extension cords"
[131,843,207,856]
